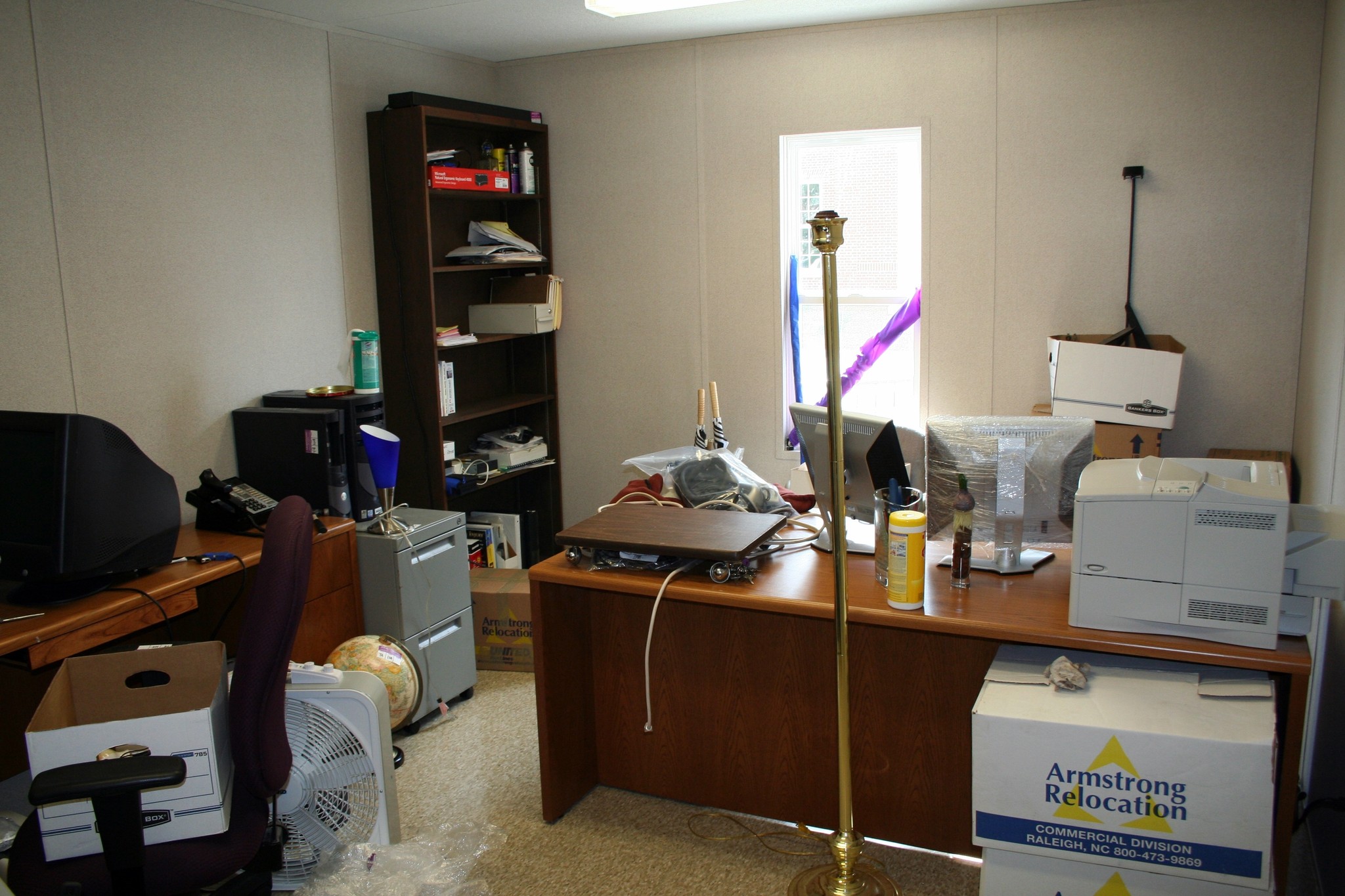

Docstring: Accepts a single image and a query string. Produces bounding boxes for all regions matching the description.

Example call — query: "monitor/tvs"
[788,402,925,554]
[926,416,1095,575]
[0,410,182,606]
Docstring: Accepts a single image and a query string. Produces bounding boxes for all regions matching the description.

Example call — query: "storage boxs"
[427,165,509,192]
[1207,448,1292,498]
[1047,332,1188,429]
[970,650,1278,896]
[1093,421,1162,461]
[467,567,533,674]
[25,639,235,859]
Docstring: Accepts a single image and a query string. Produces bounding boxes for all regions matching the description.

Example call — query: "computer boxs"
[231,406,353,523]
[261,389,388,523]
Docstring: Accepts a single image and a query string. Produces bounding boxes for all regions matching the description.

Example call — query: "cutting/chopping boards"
[554,504,788,563]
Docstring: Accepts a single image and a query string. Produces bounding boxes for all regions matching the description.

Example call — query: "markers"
[905,494,919,511]
[888,478,899,512]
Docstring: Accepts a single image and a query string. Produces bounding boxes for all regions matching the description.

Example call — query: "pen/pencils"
[883,507,888,532]
[898,486,904,511]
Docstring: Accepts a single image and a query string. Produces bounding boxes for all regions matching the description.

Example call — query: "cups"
[874,487,924,588]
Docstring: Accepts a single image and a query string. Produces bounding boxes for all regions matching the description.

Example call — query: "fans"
[272,667,402,892]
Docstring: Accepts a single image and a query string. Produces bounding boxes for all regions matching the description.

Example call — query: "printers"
[1067,454,1345,651]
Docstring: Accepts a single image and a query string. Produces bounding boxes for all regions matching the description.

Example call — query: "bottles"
[531,112,541,124]
[504,144,520,194]
[951,474,976,589]
[518,142,536,194]
[351,328,379,395]
[477,140,500,171]
[888,510,927,610]
[492,146,506,171]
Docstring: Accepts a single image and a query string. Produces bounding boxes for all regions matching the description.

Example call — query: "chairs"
[7,495,312,896]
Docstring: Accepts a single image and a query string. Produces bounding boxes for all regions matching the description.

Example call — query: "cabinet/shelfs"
[354,507,478,734]
[366,107,563,573]
[0,516,365,669]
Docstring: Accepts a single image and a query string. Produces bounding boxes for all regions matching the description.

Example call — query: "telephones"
[185,468,279,534]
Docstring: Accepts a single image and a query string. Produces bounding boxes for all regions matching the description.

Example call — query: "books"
[489,273,564,331]
[438,360,456,417]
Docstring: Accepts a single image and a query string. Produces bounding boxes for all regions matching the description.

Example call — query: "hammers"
[312,508,329,533]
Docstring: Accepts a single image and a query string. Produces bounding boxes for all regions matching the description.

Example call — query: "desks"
[528,513,1320,826]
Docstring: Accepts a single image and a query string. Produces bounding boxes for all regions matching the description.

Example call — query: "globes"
[323,634,423,776]
